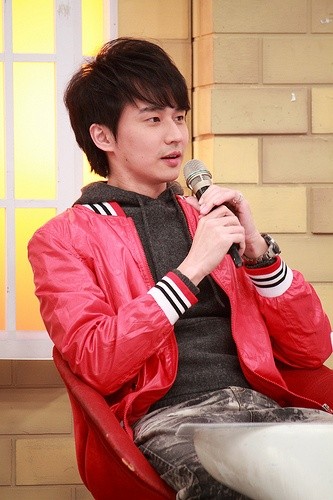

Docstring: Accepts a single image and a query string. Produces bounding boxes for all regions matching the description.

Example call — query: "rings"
[233,193,243,204]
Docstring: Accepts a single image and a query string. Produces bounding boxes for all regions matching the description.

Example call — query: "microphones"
[183,159,243,268]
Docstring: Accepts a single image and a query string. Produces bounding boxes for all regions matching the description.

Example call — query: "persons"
[27,39,332,500]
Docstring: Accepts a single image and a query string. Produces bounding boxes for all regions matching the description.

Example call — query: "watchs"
[242,234,281,264]
